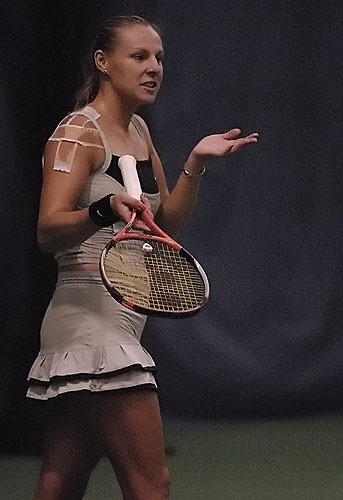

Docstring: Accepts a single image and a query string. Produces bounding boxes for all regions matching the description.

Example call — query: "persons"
[26,12,259,498]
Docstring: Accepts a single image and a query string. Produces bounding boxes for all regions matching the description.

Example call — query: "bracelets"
[89,194,122,228]
[180,163,206,179]
